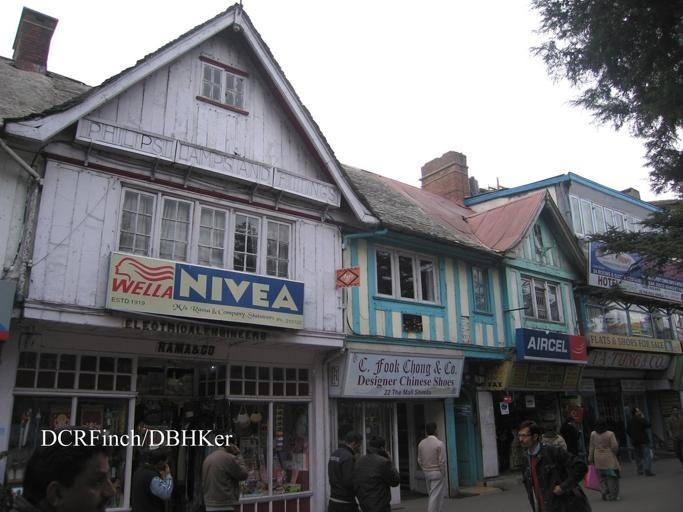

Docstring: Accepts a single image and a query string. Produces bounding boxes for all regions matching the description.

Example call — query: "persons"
[325,430,363,511]
[0,423,116,512]
[350,434,400,512]
[508,423,540,512]
[586,418,621,503]
[131,447,173,512]
[200,430,249,512]
[559,418,579,459]
[626,407,655,477]
[664,406,681,465]
[538,413,566,450]
[493,411,514,470]
[573,423,587,463]
[515,419,593,512]
[416,420,446,512]
[282,408,307,453]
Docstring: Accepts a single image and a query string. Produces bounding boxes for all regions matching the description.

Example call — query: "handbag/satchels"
[552,446,594,512]
[584,462,606,494]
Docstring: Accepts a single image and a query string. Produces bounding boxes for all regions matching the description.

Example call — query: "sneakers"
[637,470,657,476]
